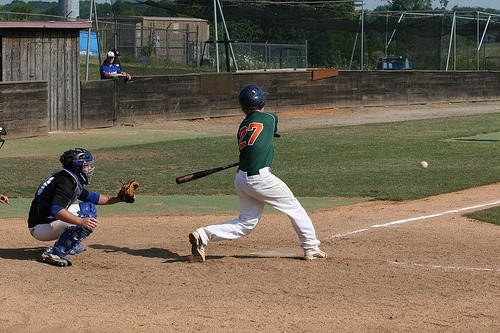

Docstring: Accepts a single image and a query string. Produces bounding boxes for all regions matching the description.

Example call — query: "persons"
[28,148,125,267]
[188,84,327,263]
[0,123,9,204]
[100,49,131,80]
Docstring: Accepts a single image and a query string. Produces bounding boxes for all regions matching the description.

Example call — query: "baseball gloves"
[117,181,140,204]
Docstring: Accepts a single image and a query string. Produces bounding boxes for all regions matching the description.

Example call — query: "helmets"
[0,124,7,149]
[239,84,269,107]
[60,148,94,185]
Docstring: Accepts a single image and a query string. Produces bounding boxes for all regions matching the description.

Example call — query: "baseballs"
[420,161,428,168]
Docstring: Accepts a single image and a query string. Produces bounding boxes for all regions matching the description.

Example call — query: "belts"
[236,166,271,177]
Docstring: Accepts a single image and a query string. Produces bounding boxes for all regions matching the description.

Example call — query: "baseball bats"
[176,162,240,185]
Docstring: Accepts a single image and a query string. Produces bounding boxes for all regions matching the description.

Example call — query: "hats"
[107,49,120,58]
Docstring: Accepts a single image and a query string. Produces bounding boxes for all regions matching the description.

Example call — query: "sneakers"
[70,243,85,254]
[189,230,205,262]
[41,251,71,266]
[304,249,328,260]
